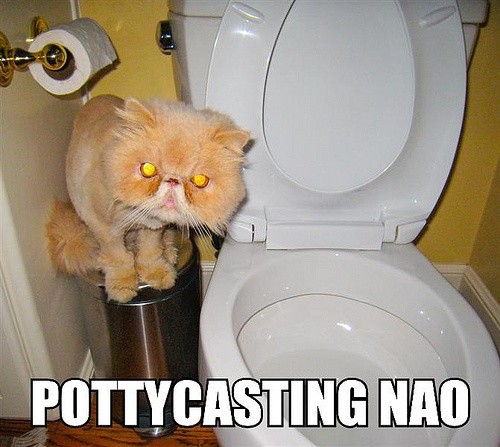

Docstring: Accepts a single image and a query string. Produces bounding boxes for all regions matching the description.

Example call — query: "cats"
[42,93,255,307]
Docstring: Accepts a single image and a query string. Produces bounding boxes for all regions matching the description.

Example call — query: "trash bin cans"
[77,227,202,438]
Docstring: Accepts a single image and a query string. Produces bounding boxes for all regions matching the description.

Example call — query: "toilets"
[155,1,500,447]
